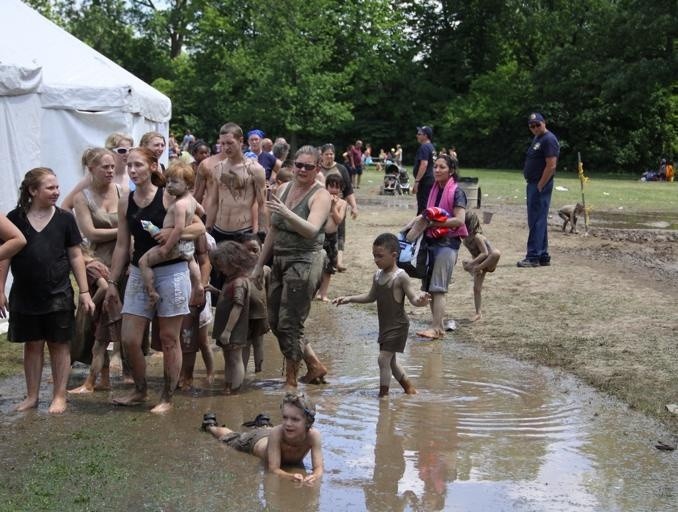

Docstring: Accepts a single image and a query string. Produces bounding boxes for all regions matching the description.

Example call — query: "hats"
[416,125,432,136]
[527,113,545,124]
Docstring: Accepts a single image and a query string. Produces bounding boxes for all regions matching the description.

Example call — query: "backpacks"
[396,228,434,278]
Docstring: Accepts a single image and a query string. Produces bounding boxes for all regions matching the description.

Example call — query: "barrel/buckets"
[483,211,494,223]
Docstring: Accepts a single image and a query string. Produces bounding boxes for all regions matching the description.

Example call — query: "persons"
[659,158,667,181]
[558,202,584,234]
[515,112,560,267]
[202,390,323,484]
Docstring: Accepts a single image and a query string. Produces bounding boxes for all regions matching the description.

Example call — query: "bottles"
[140,220,163,244]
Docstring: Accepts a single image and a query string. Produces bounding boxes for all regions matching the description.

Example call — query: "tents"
[0,0,172,298]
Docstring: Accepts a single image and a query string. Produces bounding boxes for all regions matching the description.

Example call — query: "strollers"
[378,163,412,198]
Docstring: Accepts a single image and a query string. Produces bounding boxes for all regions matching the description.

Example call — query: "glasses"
[415,133,425,136]
[529,123,544,129]
[110,146,134,155]
[292,160,318,172]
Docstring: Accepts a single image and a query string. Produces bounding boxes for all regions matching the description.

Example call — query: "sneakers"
[540,255,551,268]
[399,215,429,242]
[445,319,456,331]
[463,260,476,276]
[517,257,540,269]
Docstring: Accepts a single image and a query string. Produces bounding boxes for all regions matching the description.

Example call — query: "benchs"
[362,156,396,171]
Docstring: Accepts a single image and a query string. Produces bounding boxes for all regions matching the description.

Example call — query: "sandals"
[201,411,217,433]
[241,413,275,432]
